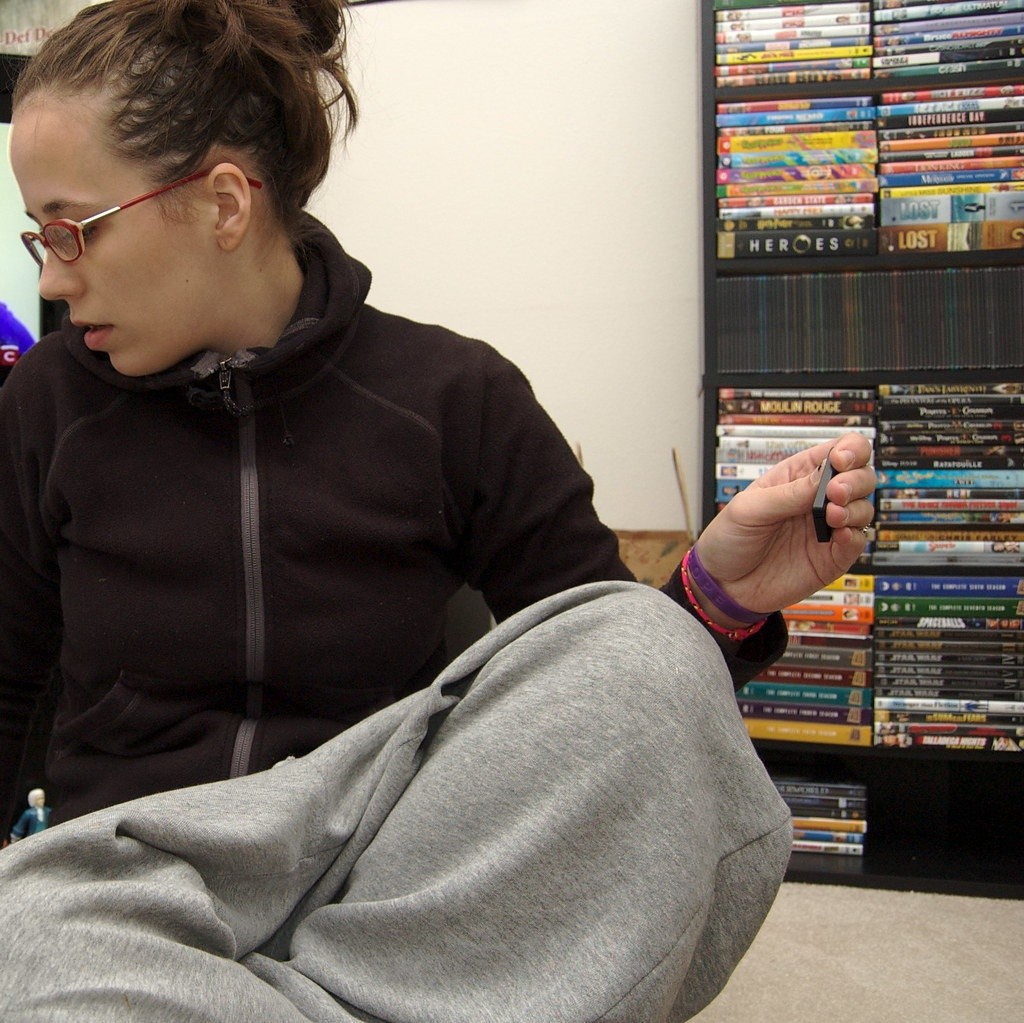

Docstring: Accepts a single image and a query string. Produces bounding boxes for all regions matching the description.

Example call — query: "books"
[716,2,1022,257]
[713,369,1024,857]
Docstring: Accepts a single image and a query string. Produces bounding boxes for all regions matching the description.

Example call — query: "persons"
[12,789,52,841]
[0,0,877,1023]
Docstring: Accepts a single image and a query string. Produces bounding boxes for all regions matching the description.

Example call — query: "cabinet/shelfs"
[701,0,1024,900]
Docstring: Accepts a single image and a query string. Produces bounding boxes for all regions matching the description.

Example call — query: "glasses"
[20,170,263,268]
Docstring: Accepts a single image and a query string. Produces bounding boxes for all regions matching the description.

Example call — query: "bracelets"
[682,550,767,639]
